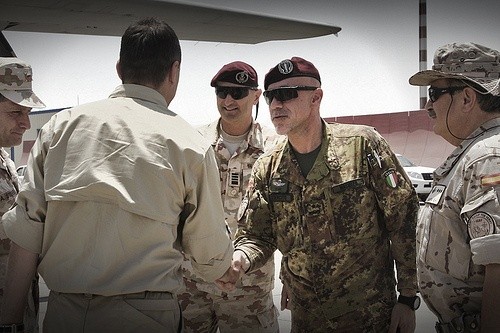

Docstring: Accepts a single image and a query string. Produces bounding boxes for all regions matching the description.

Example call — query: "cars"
[395,153,436,202]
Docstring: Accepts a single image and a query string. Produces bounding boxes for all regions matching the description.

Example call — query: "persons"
[0,57,46,333]
[215,57,421,333]
[0,17,239,333]
[408,42,500,333]
[176,61,281,333]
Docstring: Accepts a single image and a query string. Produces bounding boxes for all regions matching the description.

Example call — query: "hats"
[210,61,259,88]
[264,56,321,91]
[0,57,46,108]
[408,43,500,99]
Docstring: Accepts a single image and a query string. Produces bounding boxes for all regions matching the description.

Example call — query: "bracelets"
[0,322,24,333]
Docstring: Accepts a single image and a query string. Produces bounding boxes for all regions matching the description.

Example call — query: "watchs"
[398,294,420,311]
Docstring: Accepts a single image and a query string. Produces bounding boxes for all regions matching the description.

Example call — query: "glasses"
[263,85,319,105]
[215,87,258,100]
[428,85,474,104]
